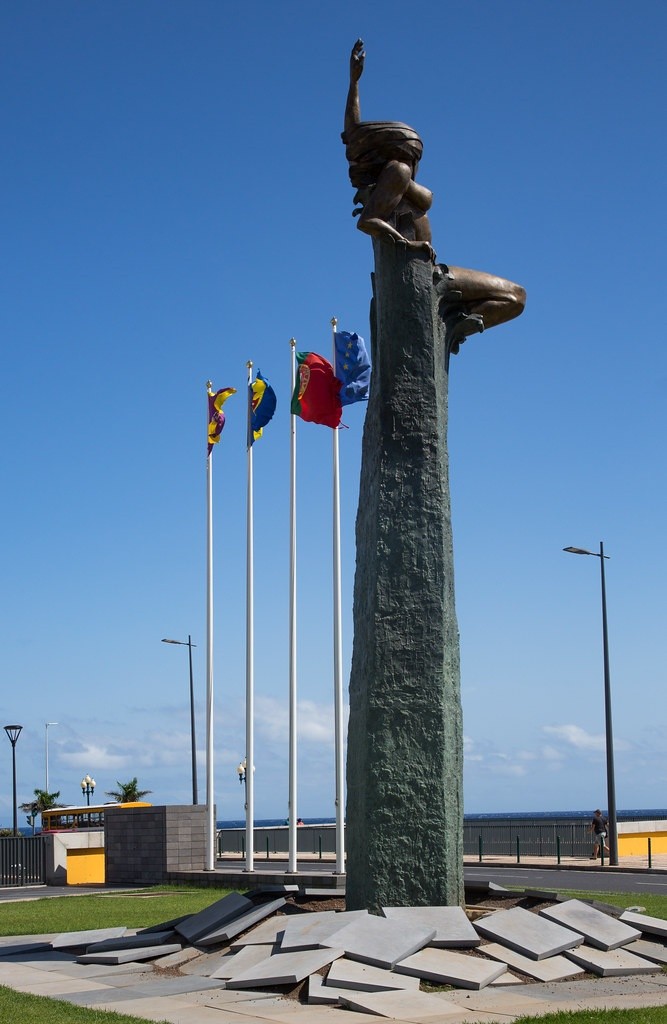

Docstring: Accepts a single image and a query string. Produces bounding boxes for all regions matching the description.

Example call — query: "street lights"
[564,547,618,867]
[162,639,199,804]
[4,726,23,838]
[237,757,255,810]
[46,722,60,797]
[82,774,95,826]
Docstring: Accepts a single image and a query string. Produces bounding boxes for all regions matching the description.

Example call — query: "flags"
[207,387,237,455]
[334,331,372,406]
[291,351,343,428]
[247,368,277,445]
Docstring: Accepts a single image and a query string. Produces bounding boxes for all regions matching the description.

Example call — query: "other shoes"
[589,857,597,860]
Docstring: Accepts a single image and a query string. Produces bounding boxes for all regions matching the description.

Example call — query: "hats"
[297,818,303,822]
[593,809,602,817]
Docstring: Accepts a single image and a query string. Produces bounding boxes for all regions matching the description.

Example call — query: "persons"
[286,818,304,826]
[586,809,609,860]
